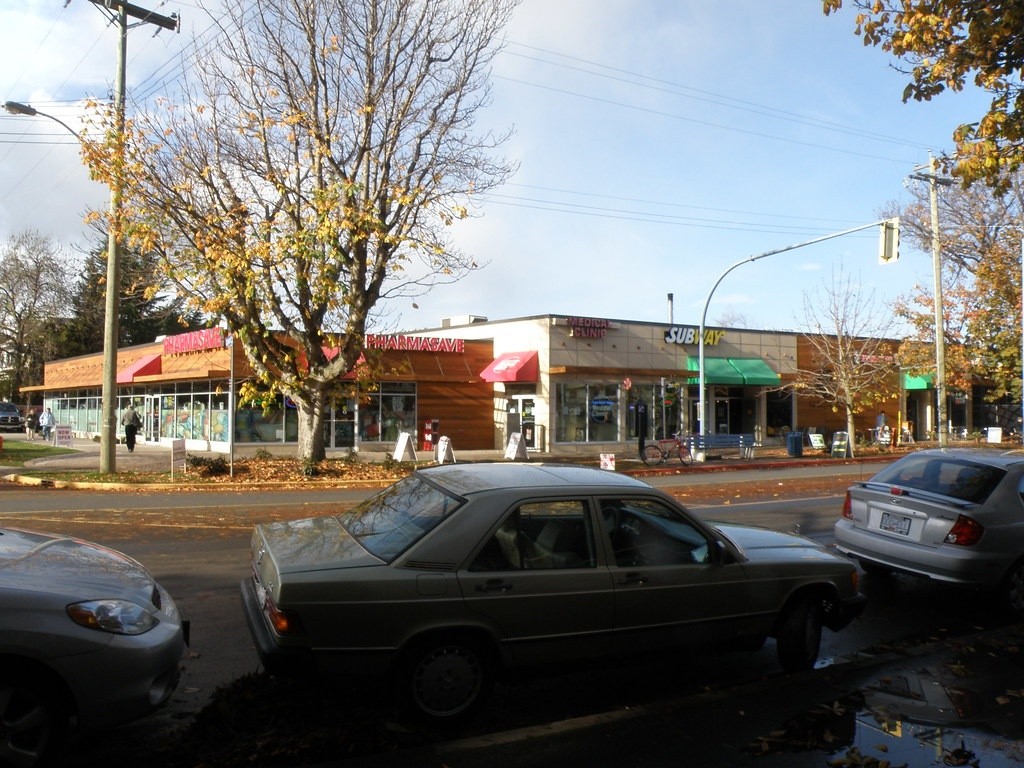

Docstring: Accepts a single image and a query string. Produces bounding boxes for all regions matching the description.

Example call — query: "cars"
[829,446,1024,608]
[234,461,863,734]
[0,402,22,432]
[0,524,197,768]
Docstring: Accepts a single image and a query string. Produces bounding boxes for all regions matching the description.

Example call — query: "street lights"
[3,1,178,479]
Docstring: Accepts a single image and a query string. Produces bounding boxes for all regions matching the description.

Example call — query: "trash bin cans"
[788,431,803,456]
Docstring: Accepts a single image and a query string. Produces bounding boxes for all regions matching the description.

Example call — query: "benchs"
[686,433,755,461]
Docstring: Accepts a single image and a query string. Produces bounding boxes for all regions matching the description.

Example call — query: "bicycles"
[640,431,693,467]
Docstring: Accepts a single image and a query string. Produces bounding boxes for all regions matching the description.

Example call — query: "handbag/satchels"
[133,413,143,428]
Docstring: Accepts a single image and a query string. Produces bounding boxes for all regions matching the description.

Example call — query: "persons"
[25,408,55,442]
[121,405,141,452]
[874,410,887,438]
